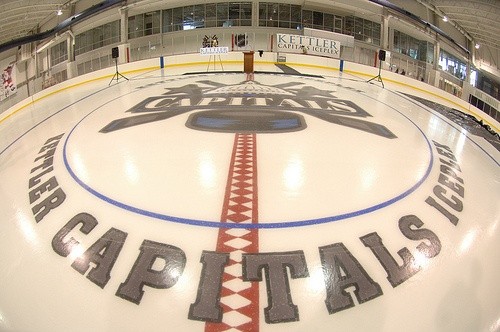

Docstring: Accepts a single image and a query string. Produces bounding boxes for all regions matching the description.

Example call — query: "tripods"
[367,60,384,88]
[109,58,128,85]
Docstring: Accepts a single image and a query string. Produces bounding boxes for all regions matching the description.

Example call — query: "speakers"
[112,47,119,58]
[379,50,385,61]
